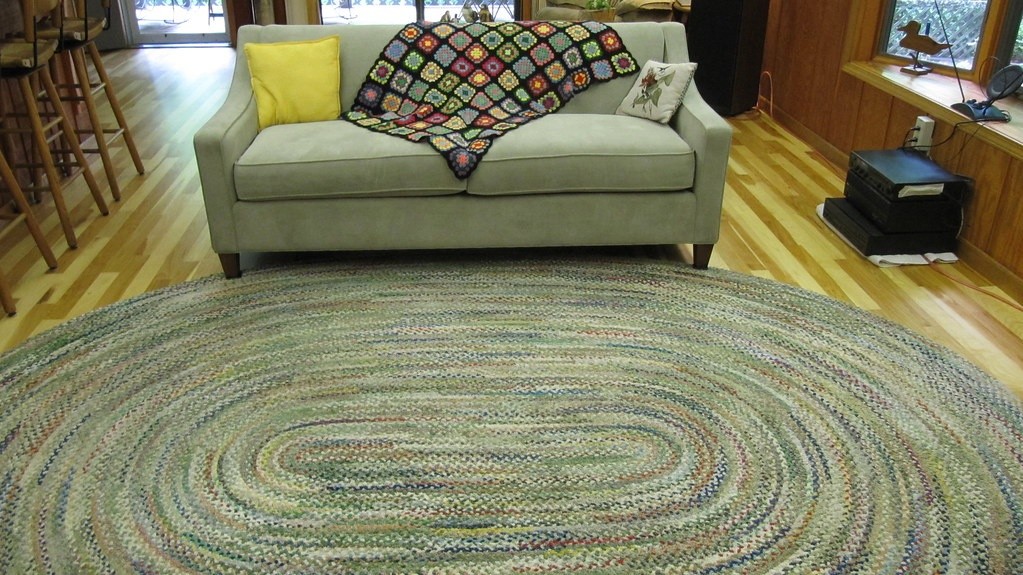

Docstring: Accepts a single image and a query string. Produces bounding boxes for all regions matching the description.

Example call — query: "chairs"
[0,0,144,313]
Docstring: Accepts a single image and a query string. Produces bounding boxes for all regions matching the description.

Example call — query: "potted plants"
[585,0,617,22]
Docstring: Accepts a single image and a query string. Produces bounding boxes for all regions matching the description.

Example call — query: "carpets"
[0,260,1023,575]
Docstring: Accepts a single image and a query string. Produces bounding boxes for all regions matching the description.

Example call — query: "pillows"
[245,34,341,129]
[615,59,698,123]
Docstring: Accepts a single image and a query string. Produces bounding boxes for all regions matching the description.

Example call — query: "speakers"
[685,0,771,117]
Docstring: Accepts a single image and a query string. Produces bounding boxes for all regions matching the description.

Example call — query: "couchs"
[193,22,732,279]
